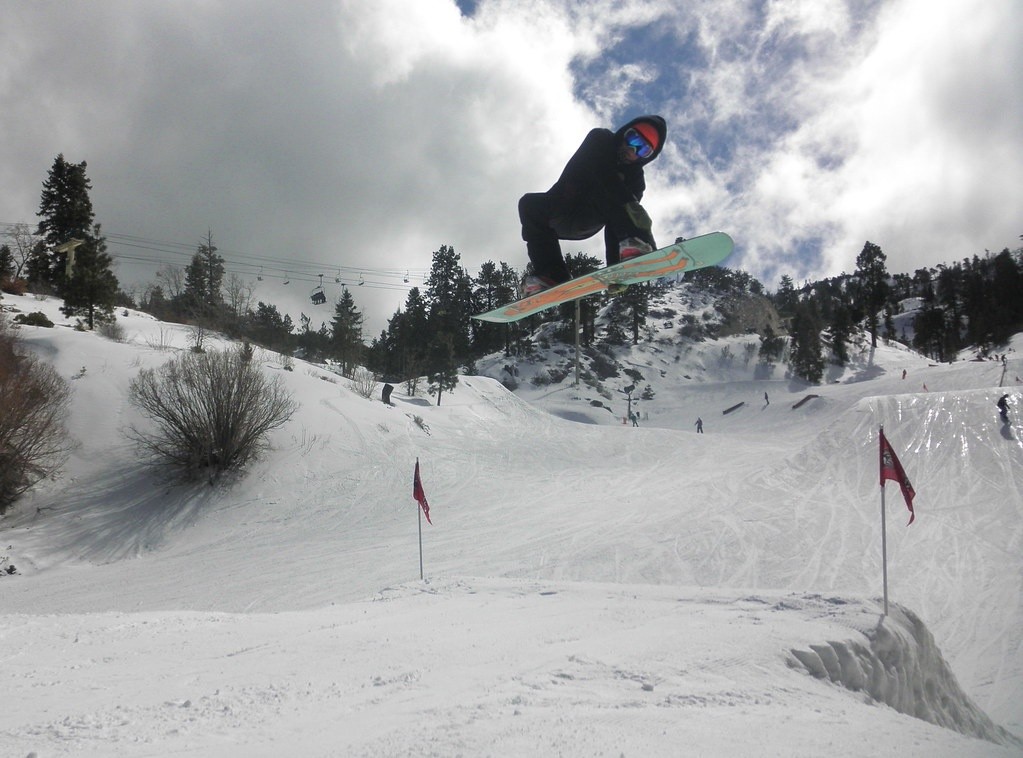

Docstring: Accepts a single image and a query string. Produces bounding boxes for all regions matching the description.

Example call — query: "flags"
[413,460,433,525]
[879,429,917,526]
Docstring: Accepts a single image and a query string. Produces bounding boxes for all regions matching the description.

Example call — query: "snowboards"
[467,231,735,324]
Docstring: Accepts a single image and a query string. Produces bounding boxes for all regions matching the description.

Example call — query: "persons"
[765,392,769,405]
[1001,354,1007,366]
[997,394,1010,416]
[995,354,999,361]
[902,369,906,379]
[694,417,703,434]
[518,116,666,296]
[631,413,639,427]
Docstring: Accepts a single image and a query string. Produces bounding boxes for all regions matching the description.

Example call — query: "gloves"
[607,284,628,294]
[625,200,652,230]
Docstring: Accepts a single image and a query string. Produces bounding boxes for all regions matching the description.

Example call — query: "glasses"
[624,128,654,159]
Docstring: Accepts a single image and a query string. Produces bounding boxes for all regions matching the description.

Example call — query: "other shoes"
[518,273,572,298]
[619,237,653,262]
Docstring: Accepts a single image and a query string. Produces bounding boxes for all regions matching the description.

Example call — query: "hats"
[632,122,660,151]
